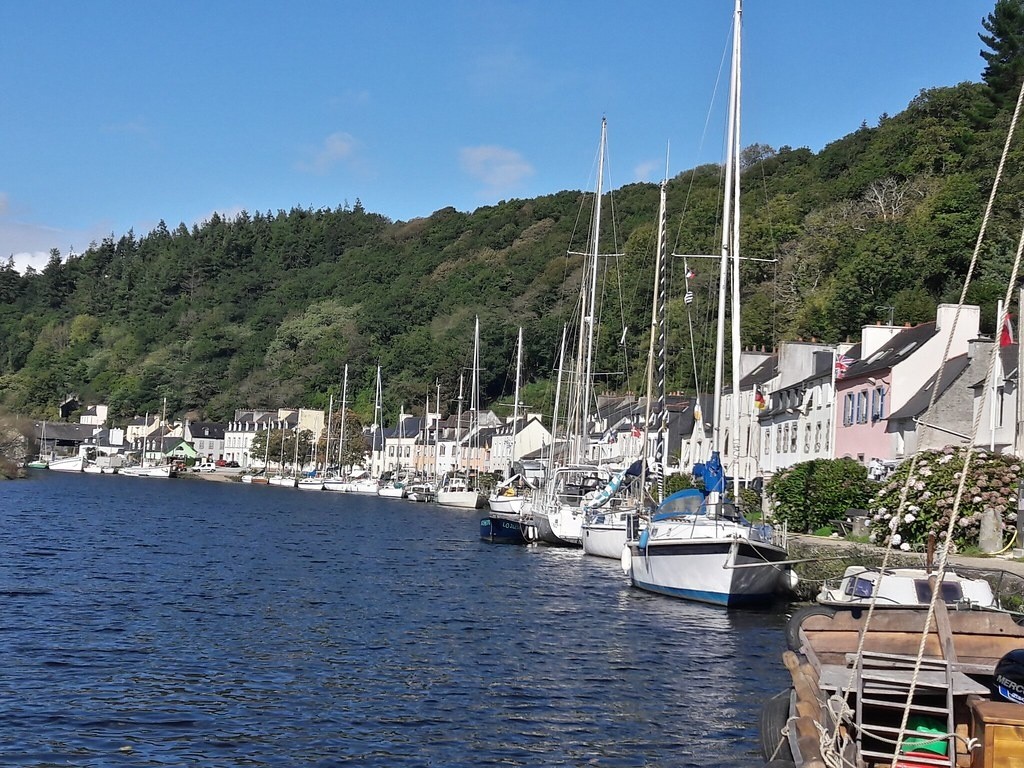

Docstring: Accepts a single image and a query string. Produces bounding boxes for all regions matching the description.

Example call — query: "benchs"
[828,508,868,537]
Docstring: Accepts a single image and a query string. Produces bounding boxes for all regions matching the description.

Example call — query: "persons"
[869,457,887,481]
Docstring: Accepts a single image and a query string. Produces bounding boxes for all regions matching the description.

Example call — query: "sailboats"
[619,1,799,610]
[25,398,173,478]
[761,73,1024,768]
[241,116,669,561]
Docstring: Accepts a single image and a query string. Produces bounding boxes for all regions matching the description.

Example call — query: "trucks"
[167,456,187,472]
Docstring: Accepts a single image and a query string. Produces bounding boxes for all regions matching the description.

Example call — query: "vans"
[190,462,216,473]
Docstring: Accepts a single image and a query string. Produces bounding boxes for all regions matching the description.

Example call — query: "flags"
[997,313,1013,348]
[752,387,766,410]
[632,426,641,437]
[608,428,618,443]
[832,353,857,380]
[685,267,697,281]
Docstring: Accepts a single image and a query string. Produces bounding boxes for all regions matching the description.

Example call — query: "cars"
[225,461,238,467]
[216,460,227,466]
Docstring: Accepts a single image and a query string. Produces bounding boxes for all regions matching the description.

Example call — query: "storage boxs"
[969,700,1024,768]
